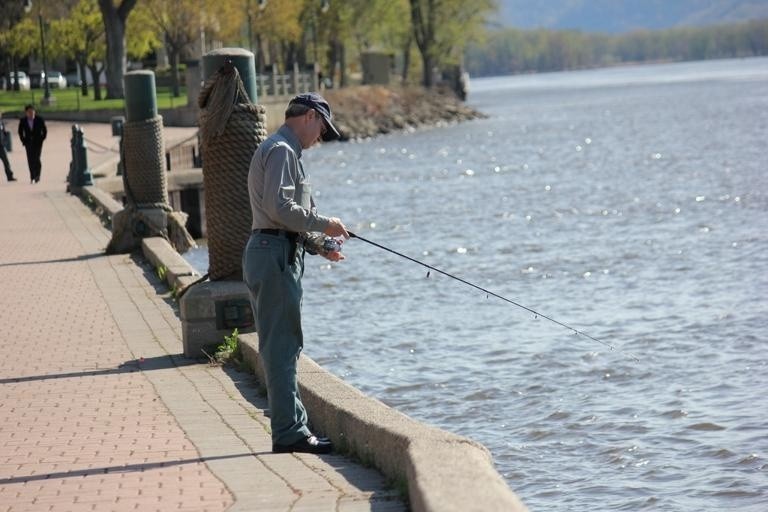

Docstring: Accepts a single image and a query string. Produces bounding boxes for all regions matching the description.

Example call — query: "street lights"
[21,0,50,99]
[246,0,268,52]
[309,1,330,62]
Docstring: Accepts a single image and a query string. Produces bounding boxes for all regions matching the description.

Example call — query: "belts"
[253,228,299,241]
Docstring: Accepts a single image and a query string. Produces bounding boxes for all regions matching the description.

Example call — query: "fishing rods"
[320,231,641,362]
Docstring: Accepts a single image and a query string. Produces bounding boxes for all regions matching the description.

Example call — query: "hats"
[288,92,341,142]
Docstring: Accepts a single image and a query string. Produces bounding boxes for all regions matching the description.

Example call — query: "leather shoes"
[272,433,333,453]
[317,437,329,441]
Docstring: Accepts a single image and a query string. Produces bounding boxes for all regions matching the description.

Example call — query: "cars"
[39,71,68,90]
[2,72,31,91]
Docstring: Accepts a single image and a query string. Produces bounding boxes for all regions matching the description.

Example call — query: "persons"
[16,102,47,184]
[0,108,16,182]
[239,91,351,455]
